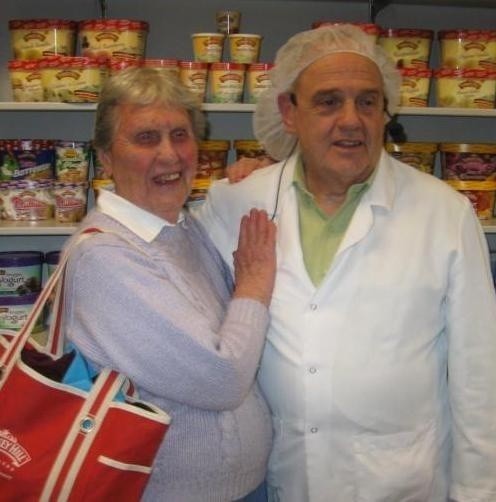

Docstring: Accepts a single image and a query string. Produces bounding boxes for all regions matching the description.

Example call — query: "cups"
[53,140,117,223]
[5,8,276,103]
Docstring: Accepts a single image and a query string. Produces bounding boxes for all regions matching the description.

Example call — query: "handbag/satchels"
[0,328,173,502]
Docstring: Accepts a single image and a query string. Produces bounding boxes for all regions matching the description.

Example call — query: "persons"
[48,65,280,500]
[190,21,495,502]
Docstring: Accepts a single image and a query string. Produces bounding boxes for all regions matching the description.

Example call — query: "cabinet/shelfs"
[0,100,494,351]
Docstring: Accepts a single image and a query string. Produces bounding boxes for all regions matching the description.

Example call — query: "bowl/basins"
[312,17,496,108]
[382,142,496,226]
[0,248,66,334]
[178,137,260,214]
